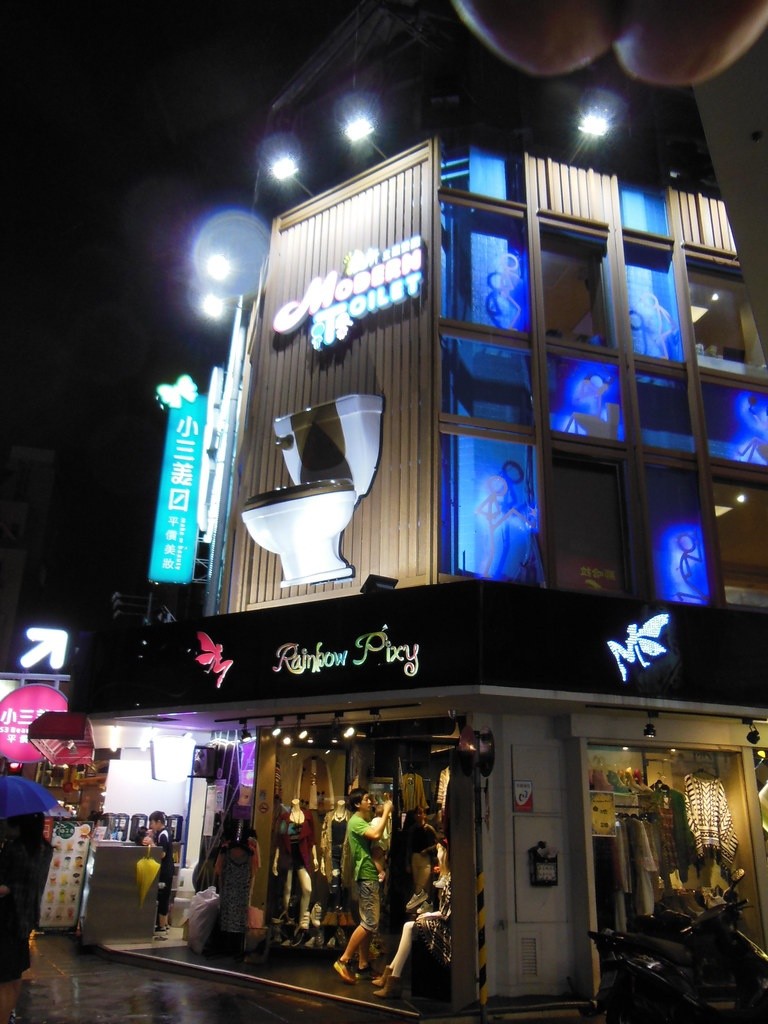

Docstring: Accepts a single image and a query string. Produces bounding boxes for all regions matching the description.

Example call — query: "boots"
[373,975,403,999]
[371,965,394,987]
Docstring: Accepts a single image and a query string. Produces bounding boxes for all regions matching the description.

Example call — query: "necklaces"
[335,812,346,822]
[292,811,300,834]
[421,823,426,827]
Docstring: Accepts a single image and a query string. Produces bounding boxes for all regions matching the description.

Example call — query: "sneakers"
[406,889,428,909]
[417,900,434,914]
[299,912,309,929]
[311,903,322,927]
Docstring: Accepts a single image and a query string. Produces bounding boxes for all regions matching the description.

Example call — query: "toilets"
[237,395,387,588]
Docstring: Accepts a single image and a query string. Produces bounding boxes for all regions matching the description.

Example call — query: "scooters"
[582,869,768,1024]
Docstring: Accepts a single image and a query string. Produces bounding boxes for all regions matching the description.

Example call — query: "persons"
[371,839,451,998]
[135,826,154,846]
[272,799,319,916]
[320,799,353,909]
[0,813,53,1024]
[332,788,394,983]
[143,811,174,935]
[409,807,438,895]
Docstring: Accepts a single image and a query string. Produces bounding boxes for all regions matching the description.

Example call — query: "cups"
[44,839,85,921]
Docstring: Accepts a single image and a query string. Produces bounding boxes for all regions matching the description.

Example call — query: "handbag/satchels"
[341,832,353,888]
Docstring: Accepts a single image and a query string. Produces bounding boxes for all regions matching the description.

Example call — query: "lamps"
[566,117,610,165]
[643,711,658,737]
[238,720,252,741]
[270,158,316,199]
[345,118,390,161]
[753,750,767,772]
[741,717,760,744]
[267,710,383,747]
[360,573,398,594]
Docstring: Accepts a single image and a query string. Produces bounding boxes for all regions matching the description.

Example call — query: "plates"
[79,824,91,835]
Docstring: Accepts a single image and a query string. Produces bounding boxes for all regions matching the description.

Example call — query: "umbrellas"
[0,776,58,818]
[137,845,161,909]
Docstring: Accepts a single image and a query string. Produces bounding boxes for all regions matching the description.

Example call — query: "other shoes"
[359,963,371,971]
[347,912,355,924]
[328,912,338,925]
[165,924,170,929]
[156,927,166,930]
[270,924,347,950]
[338,913,346,925]
[378,871,386,882]
[333,962,355,983]
[322,912,332,925]
[8,1012,16,1024]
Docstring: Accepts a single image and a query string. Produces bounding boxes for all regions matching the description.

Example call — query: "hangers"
[612,763,720,821]
[402,764,423,775]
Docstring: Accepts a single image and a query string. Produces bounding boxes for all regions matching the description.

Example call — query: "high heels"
[590,767,654,794]
[460,725,474,776]
[479,726,495,777]
